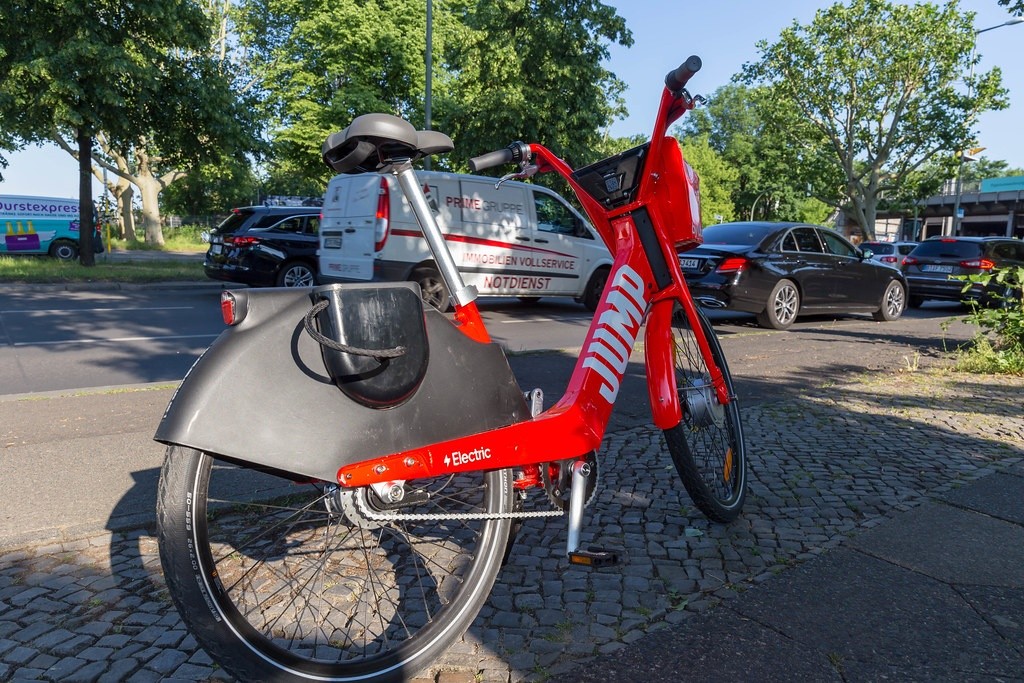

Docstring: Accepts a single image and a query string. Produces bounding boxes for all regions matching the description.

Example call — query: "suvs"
[899,236,1024,309]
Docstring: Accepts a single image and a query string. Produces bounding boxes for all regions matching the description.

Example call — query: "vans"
[315,170,616,314]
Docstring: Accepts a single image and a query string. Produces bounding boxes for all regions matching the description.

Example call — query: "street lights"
[950,18,1024,237]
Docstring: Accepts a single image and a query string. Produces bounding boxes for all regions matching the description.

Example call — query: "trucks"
[0,194,104,262]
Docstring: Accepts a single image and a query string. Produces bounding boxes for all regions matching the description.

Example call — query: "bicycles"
[152,57,746,682]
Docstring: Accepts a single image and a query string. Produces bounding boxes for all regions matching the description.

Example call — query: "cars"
[677,220,909,330]
[203,206,322,288]
[848,242,920,270]
